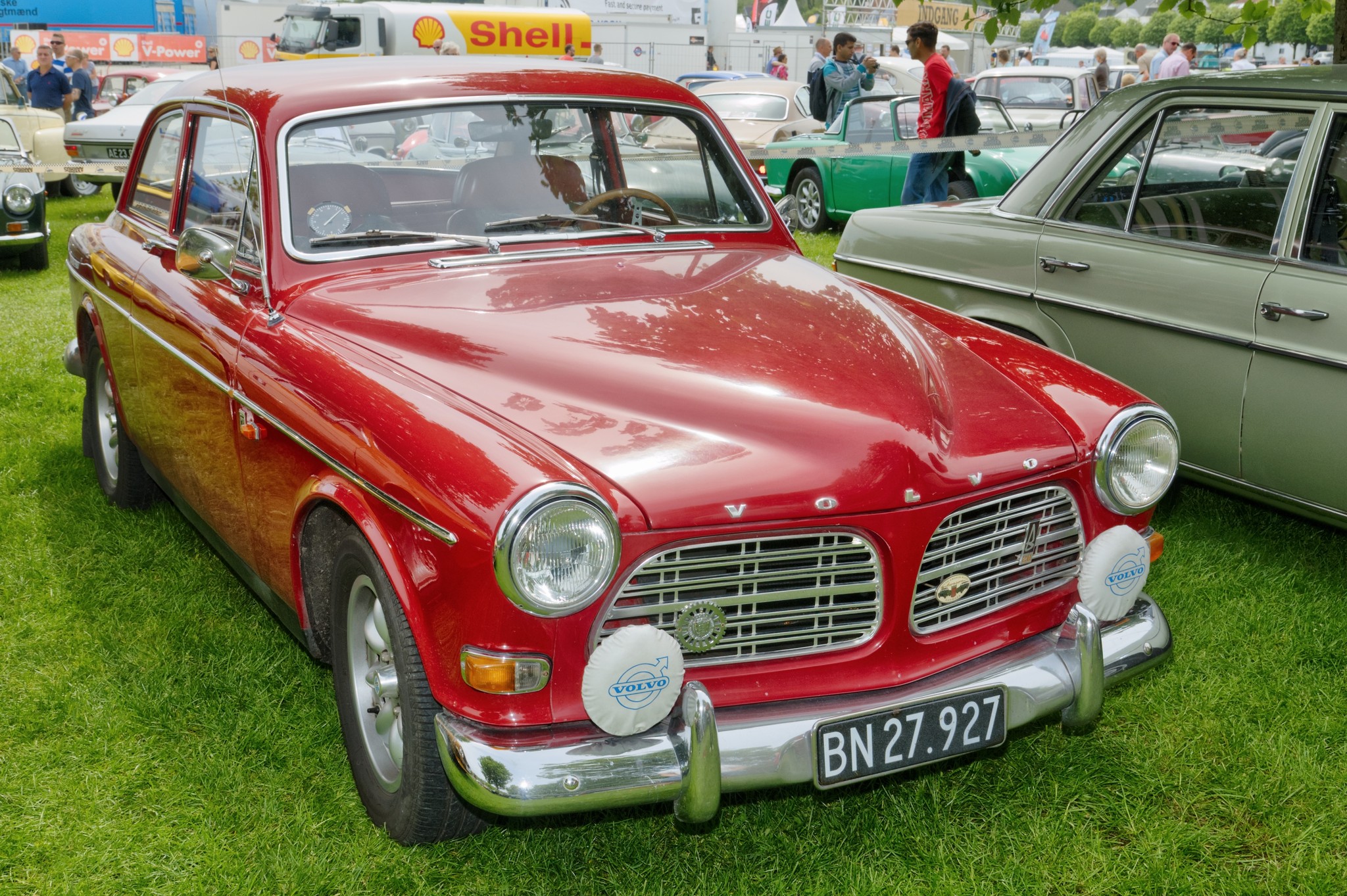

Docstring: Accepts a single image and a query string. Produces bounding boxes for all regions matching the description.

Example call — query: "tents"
[1014,40,1125,67]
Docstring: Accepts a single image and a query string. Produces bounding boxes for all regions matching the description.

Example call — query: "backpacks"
[809,65,839,122]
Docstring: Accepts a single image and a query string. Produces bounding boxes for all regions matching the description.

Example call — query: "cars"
[0,116,52,272]
[0,1,1347,232]
[60,68,216,205]
[56,0,1191,851]
[830,62,1347,543]
[640,76,826,149]
[755,89,1139,235]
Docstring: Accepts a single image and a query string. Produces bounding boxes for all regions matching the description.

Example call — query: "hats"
[773,46,783,55]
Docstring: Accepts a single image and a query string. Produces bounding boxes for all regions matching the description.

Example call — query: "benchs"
[1076,186,1291,250]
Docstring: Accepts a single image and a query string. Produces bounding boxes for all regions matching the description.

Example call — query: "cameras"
[873,62,880,69]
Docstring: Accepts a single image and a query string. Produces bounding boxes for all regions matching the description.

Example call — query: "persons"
[994,49,1032,68]
[821,32,875,133]
[0,32,100,198]
[1230,46,1256,71]
[888,43,912,58]
[706,45,720,70]
[898,22,956,208]
[806,37,833,84]
[422,39,604,65]
[940,44,960,78]
[1279,53,1324,66]
[1093,32,1197,97]
[764,46,788,81]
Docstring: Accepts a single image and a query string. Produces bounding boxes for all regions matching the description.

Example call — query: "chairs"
[286,164,392,238]
[339,29,354,42]
[456,155,600,232]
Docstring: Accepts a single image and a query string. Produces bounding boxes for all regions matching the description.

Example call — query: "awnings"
[891,25,969,51]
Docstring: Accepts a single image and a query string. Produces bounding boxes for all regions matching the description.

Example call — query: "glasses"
[51,41,63,45]
[905,35,921,45]
[66,54,78,59]
[1191,50,1195,59]
[1168,41,1180,46]
